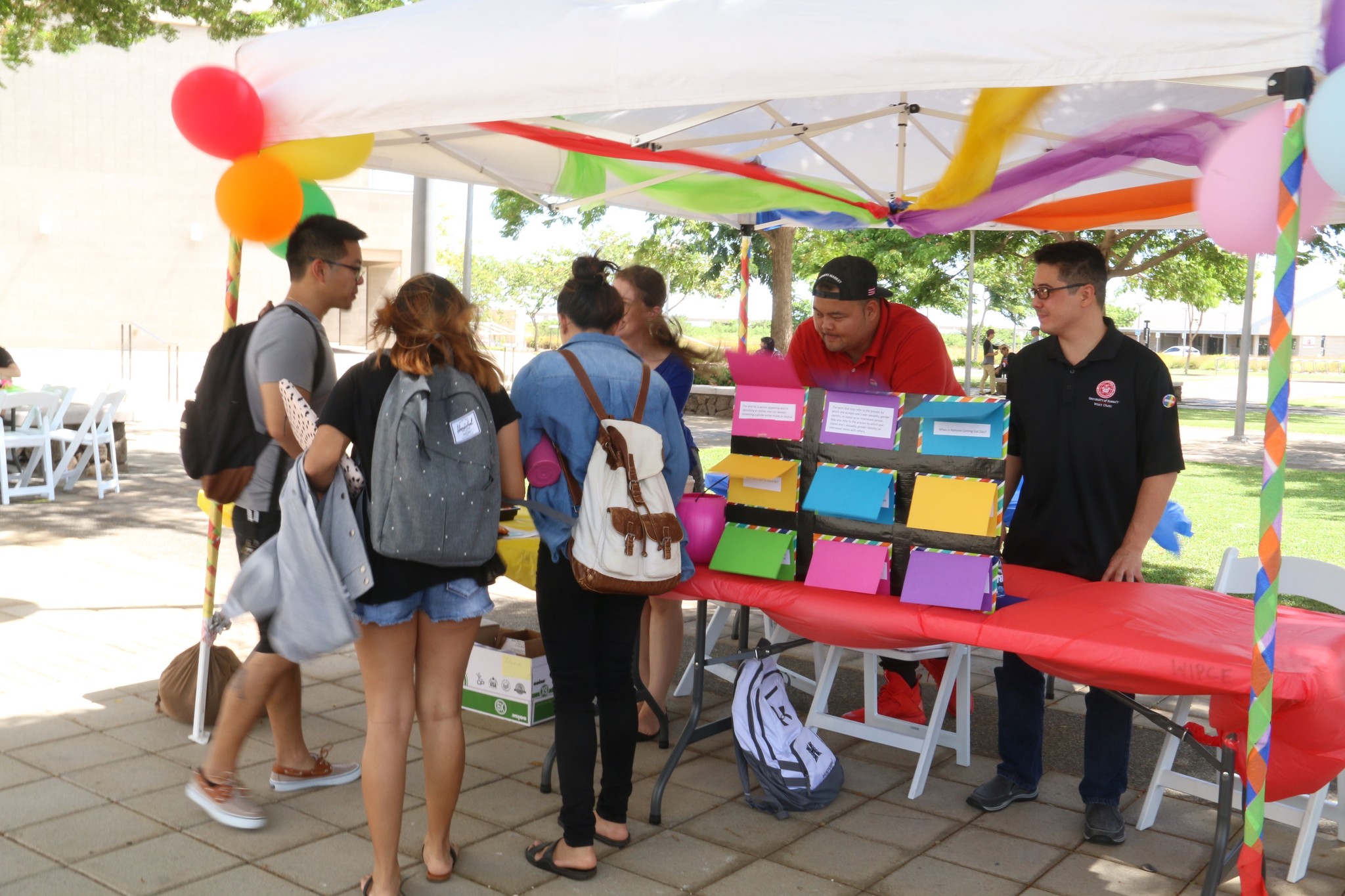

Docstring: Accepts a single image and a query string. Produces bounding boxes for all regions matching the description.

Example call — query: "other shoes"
[636,705,669,742]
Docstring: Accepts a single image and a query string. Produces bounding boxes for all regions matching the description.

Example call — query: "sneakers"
[966,773,1040,813]
[979,392,1002,397]
[919,658,973,717]
[185,765,271,830]
[269,752,361,793]
[842,670,928,726]
[1083,803,1126,844]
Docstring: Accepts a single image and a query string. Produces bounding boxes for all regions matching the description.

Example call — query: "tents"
[190,1,1345,896]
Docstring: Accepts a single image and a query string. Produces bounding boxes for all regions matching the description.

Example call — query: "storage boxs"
[459,618,560,730]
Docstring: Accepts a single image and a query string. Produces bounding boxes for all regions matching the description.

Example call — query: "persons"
[968,242,1186,845]
[1028,327,1043,344]
[0,347,20,382]
[788,255,971,720]
[978,329,1012,397]
[182,213,367,828]
[303,274,524,895]
[753,337,784,360]
[511,247,695,880]
[613,263,703,743]
[985,344,1016,395]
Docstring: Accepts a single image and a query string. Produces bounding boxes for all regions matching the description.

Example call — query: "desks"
[646,555,1345,896]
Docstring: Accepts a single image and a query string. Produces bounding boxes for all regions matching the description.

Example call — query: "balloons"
[216,157,303,244]
[1196,98,1339,255]
[260,180,336,259]
[172,67,264,162]
[259,132,376,180]
[1304,62,1345,201]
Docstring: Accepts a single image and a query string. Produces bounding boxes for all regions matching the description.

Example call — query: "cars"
[1162,346,1201,355]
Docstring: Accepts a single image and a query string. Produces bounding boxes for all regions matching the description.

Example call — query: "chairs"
[45,387,124,500]
[1131,546,1345,887]
[2,388,58,506]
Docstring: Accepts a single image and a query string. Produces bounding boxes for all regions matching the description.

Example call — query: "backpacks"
[730,637,846,820]
[543,348,683,596]
[362,347,502,568]
[180,301,326,504]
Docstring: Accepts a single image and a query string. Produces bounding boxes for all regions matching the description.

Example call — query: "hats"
[812,254,894,301]
[986,329,997,334]
[1028,326,1040,331]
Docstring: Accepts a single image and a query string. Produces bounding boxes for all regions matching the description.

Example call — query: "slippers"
[524,836,597,881]
[421,842,460,883]
[557,808,631,846]
[363,874,407,896]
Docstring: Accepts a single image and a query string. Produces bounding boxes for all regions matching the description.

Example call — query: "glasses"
[1028,283,1098,300]
[306,255,364,282]
